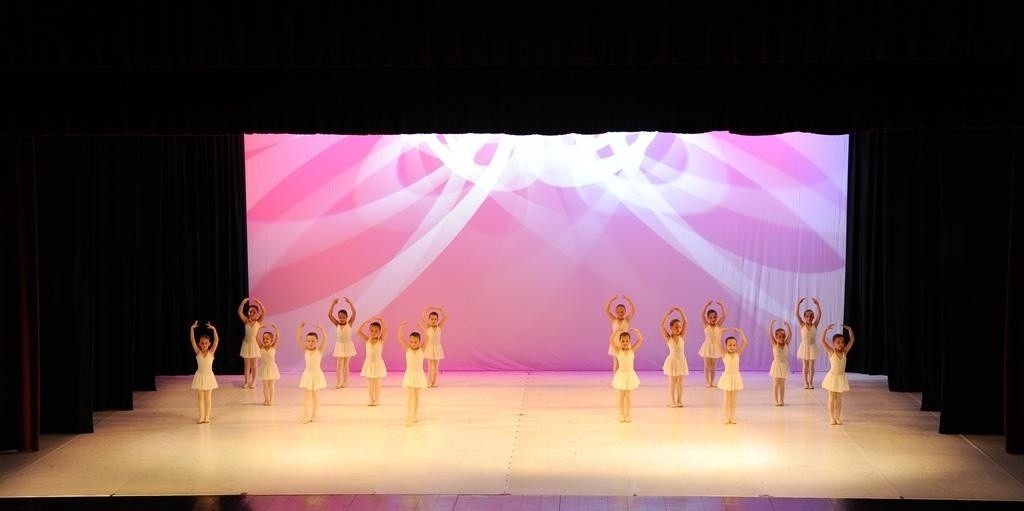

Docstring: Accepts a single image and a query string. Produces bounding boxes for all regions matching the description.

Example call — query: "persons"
[326,297,356,390]
[605,295,634,376]
[699,299,726,389]
[255,321,278,406]
[819,323,856,429]
[236,296,266,390]
[769,320,792,408]
[420,305,445,390]
[355,315,387,408]
[189,321,220,424]
[716,327,747,425]
[659,306,690,409]
[399,321,430,427]
[795,296,821,391]
[296,320,327,425]
[608,327,642,426]
